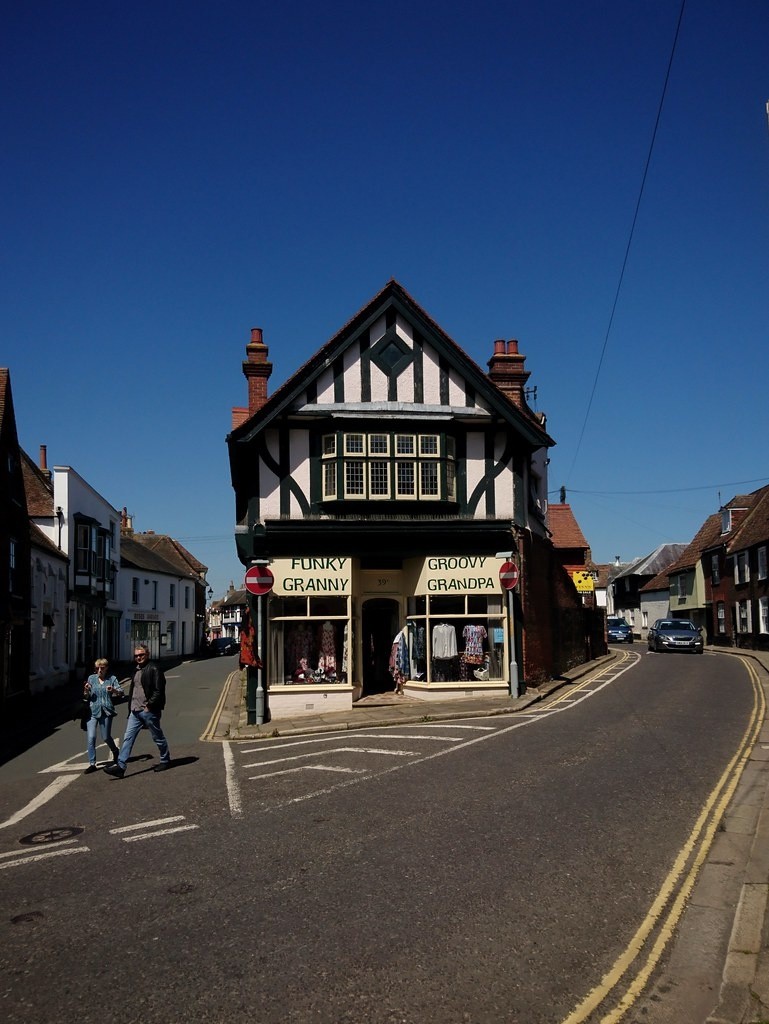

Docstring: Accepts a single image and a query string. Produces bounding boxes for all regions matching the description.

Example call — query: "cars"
[648,618,704,654]
[208,637,236,656]
[607,617,633,644]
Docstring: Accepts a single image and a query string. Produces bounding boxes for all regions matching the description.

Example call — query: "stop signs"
[498,562,519,590]
[244,566,275,595]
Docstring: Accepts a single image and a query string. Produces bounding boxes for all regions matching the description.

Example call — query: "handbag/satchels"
[75,696,92,722]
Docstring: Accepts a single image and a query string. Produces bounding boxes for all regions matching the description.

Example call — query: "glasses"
[96,667,104,670]
[135,654,145,657]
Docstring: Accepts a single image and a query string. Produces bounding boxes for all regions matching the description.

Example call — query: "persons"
[315,620,337,672]
[288,624,315,671]
[104,644,171,779]
[82,659,126,774]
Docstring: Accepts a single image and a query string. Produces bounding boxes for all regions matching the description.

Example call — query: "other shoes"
[84,766,97,774]
[154,763,171,771]
[103,766,125,777]
